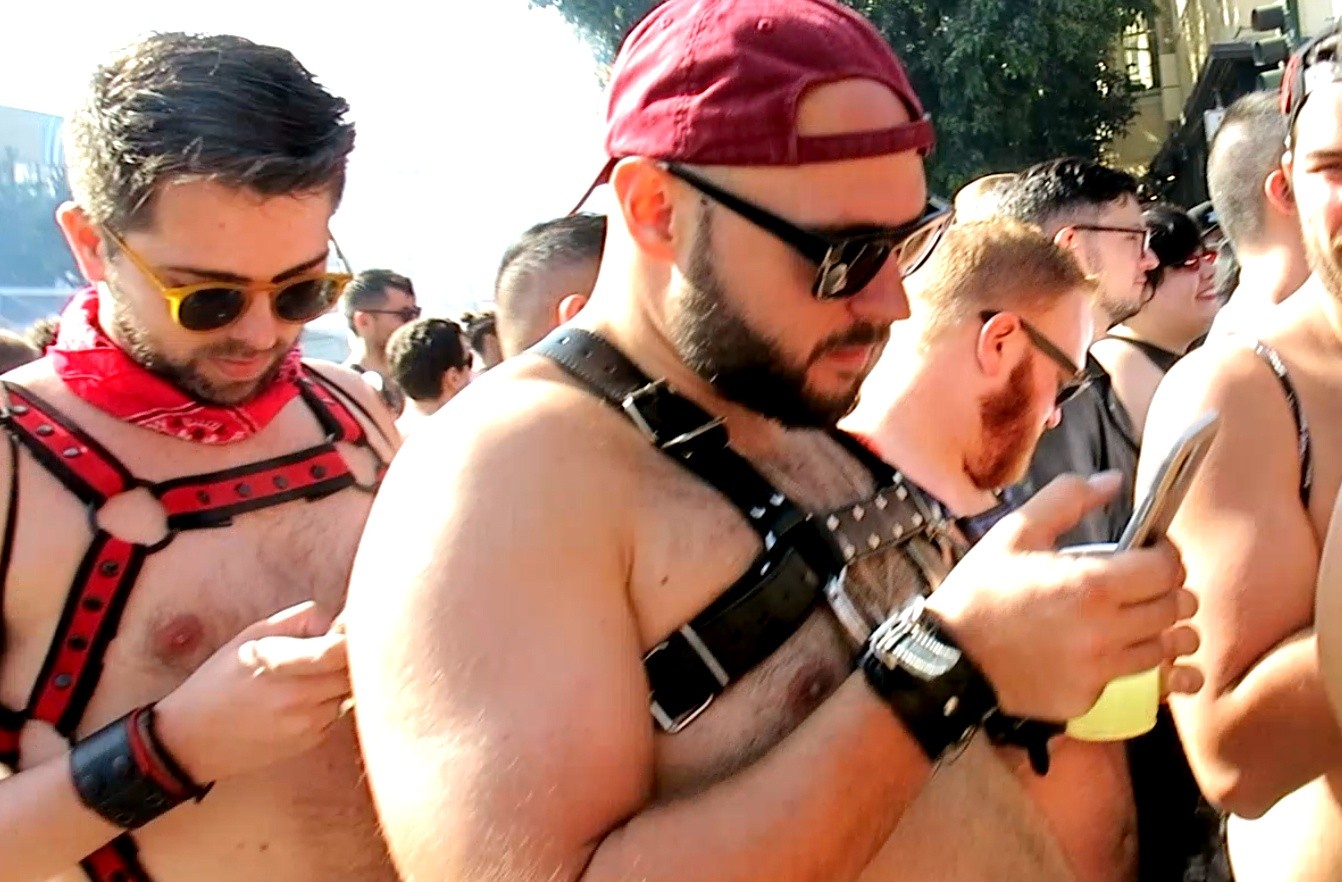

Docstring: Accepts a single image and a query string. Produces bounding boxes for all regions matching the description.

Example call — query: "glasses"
[1072,219,1151,258]
[89,210,354,334]
[455,352,473,370]
[1179,250,1219,272]
[979,308,1098,409]
[361,306,422,323]
[661,159,957,302]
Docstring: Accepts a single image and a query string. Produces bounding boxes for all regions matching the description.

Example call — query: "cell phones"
[1115,410,1225,555]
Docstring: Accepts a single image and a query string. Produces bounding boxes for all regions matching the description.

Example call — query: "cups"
[1059,542,1159,742]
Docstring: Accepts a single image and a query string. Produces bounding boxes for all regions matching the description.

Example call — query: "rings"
[338,696,354,718]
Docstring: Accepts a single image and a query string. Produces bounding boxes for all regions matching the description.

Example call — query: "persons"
[338,209,607,445]
[0,330,38,375]
[1134,24,1342,882]
[0,30,405,882]
[835,87,1313,881]
[342,2,1206,882]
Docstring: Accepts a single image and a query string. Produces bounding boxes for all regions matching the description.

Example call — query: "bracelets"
[70,700,215,831]
[852,603,997,760]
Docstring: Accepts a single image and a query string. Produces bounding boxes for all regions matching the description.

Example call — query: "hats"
[567,0,935,222]
[1278,18,1342,148]
